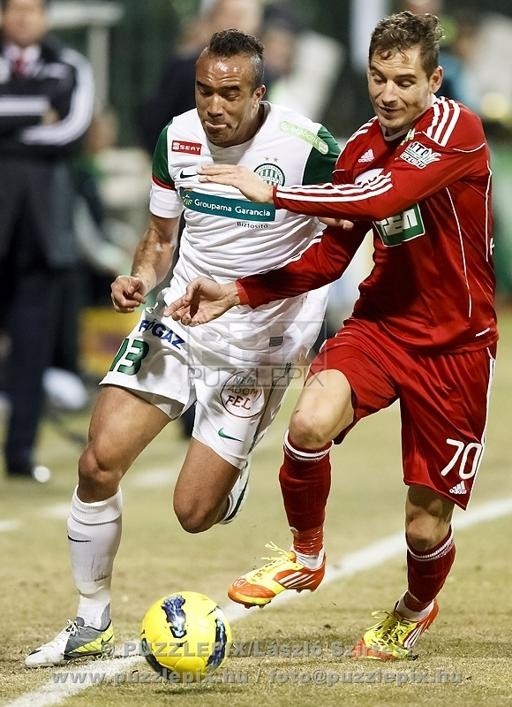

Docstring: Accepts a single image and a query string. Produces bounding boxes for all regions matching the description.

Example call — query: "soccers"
[140,591,231,682]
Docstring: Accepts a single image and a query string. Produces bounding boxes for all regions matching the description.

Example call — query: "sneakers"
[25,620,113,668]
[227,549,326,608]
[351,598,439,661]
[220,463,250,524]
[43,370,87,410]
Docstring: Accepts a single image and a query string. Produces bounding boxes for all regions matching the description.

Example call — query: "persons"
[163,11,499,661]
[25,29,355,668]
